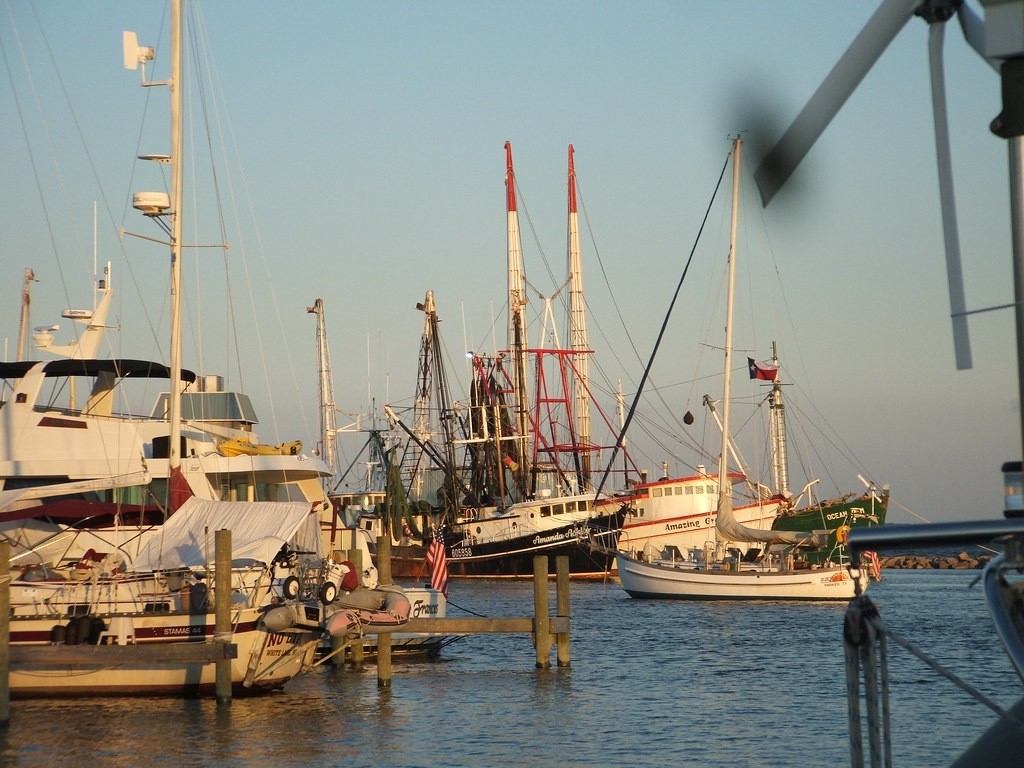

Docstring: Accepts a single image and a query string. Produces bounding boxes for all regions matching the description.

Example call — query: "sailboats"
[1,0,787,691]
[615,133,882,602]
[749,339,891,531]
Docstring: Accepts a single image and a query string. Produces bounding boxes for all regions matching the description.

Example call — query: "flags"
[424,529,448,598]
[747,357,780,380]
